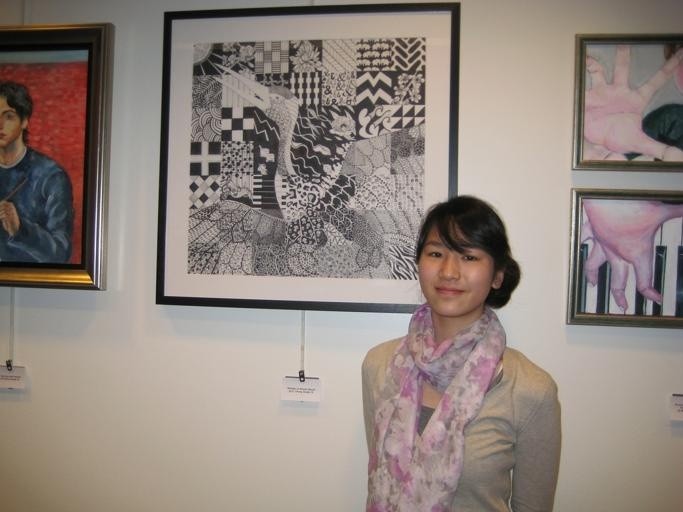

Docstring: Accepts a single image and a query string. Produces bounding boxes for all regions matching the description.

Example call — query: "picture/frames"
[0,21,115,291]
[570,32,683,172]
[564,187,683,330]
[154,1,461,314]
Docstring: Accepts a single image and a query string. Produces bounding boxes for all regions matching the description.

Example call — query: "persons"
[581,41,683,165]
[358,192,562,512]
[0,79,74,268]
[578,197,682,311]
